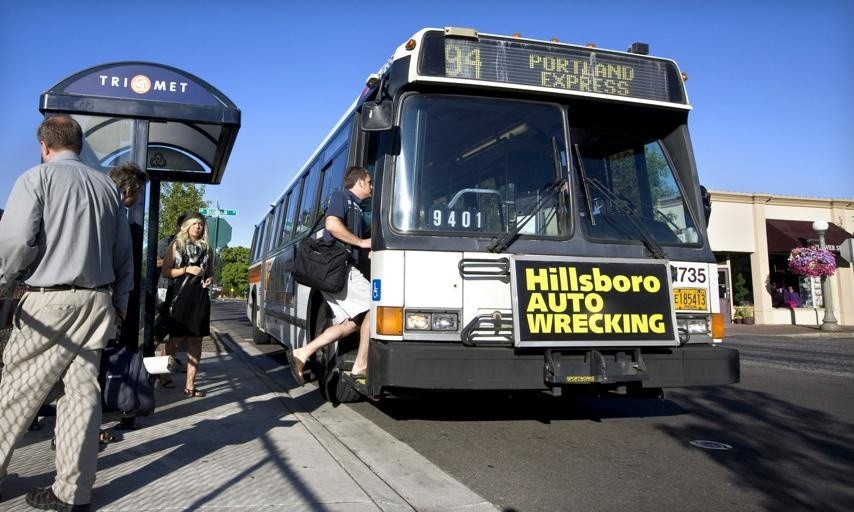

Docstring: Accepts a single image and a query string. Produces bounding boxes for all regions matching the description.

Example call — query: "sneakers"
[26,488,90,511]
[175,366,187,373]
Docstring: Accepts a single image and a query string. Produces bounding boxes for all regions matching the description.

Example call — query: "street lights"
[810,220,843,333]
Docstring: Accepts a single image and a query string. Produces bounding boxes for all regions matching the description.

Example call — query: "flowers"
[789,245,837,276]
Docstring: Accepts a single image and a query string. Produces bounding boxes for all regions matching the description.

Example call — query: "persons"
[0,115,135,512]
[152,215,189,373]
[158,213,214,397]
[285,167,373,387]
[51,163,148,449]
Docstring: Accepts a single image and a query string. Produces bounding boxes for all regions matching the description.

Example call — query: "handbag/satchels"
[291,235,351,292]
[98,348,154,414]
[158,305,174,328]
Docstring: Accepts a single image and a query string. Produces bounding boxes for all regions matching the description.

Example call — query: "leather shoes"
[350,368,368,379]
[286,349,306,386]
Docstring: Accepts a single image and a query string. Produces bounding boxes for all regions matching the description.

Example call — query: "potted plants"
[734,307,753,325]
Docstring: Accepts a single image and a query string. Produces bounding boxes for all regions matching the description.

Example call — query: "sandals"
[184,387,204,397]
[158,375,176,389]
[98,430,114,442]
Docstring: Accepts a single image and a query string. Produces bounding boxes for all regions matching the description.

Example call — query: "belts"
[25,285,80,292]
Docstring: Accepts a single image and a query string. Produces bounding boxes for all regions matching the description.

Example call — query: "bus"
[243,26,742,405]
